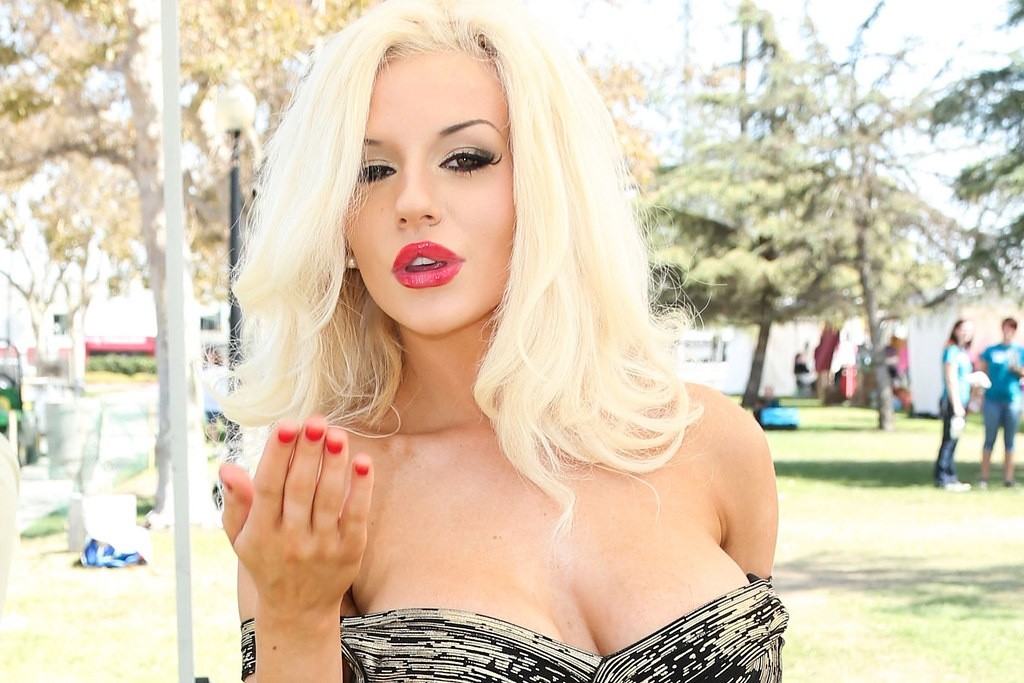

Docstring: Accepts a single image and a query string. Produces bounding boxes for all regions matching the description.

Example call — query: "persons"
[795,353,816,392]
[935,319,985,493]
[218,1,790,683]
[203,349,223,442]
[975,319,1024,488]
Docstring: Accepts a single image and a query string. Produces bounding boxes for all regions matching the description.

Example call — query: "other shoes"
[938,480,971,493]
[978,477,988,491]
[1006,479,1022,488]
